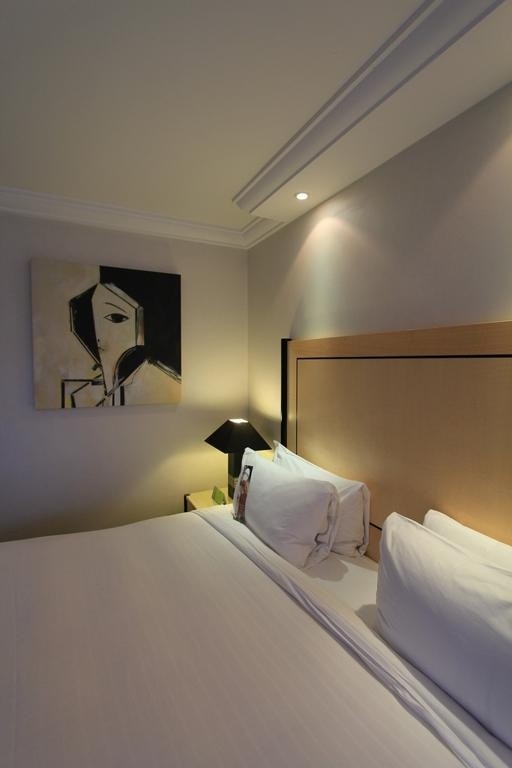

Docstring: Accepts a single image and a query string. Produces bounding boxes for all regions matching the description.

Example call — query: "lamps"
[203,419,271,500]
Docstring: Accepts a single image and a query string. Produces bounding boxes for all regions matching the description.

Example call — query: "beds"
[0,319,512,768]
